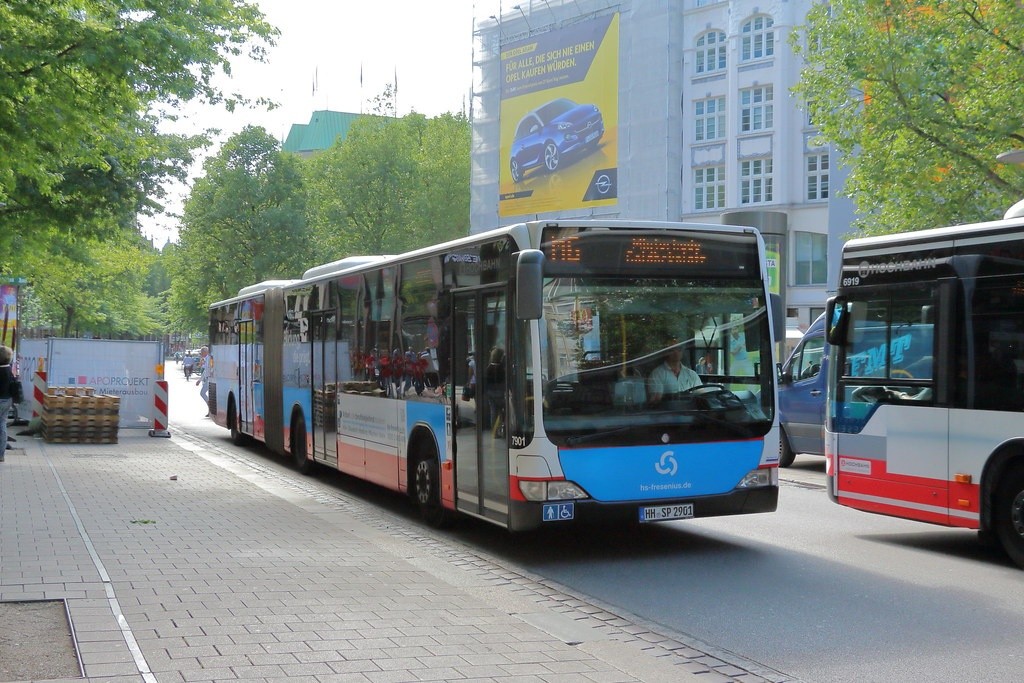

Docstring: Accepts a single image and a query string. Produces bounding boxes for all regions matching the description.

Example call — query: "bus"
[824,199,1024,572]
[208,218,788,532]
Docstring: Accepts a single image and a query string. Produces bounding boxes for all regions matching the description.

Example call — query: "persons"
[352,347,434,399]
[696,356,709,374]
[649,336,704,406]
[0,345,18,461]
[730,324,756,391]
[176,353,180,364]
[885,387,932,400]
[465,348,506,438]
[182,354,194,376]
[196,346,211,417]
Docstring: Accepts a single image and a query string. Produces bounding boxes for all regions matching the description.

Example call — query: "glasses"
[668,347,682,353]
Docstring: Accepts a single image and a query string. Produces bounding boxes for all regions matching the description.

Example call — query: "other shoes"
[6,444,11,449]
[206,413,209,417]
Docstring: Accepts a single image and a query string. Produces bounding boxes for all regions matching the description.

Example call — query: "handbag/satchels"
[10,371,24,404]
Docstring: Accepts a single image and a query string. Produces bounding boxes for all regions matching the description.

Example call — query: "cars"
[510,97,605,183]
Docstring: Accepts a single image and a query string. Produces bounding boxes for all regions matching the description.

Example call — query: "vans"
[755,304,934,468]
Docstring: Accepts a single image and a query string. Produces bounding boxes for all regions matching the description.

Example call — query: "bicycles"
[182,364,191,382]
